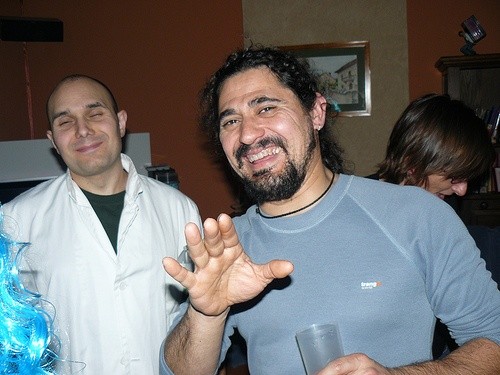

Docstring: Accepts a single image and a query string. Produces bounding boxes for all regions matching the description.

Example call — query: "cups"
[295,322,345,375]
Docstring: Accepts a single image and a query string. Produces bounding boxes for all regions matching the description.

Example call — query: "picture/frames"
[274,40,371,117]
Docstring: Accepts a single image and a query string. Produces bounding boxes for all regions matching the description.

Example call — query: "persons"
[325,70,354,96]
[156,41,500,375]
[362,92,493,200]
[0,76,206,375]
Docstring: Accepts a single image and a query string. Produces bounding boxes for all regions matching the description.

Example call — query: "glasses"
[441,169,469,184]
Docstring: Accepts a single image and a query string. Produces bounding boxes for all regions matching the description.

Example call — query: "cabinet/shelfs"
[435,54,500,226]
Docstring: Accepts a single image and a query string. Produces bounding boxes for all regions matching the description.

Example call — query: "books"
[469,104,500,194]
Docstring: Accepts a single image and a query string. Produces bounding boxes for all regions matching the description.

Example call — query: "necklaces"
[256,169,336,219]
